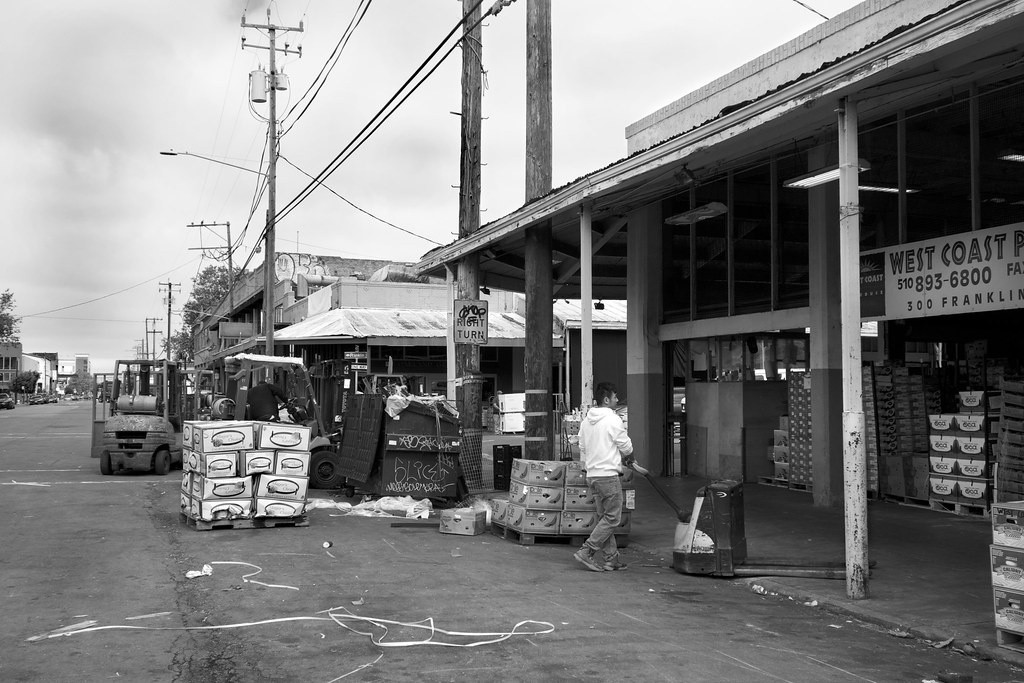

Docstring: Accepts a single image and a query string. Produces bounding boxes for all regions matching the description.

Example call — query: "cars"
[28,392,92,405]
[0,393,15,410]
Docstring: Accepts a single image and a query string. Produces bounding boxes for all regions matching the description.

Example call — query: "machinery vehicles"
[90,358,191,476]
[198,351,342,490]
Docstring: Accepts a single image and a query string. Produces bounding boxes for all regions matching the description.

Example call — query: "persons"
[246,380,290,422]
[573,381,638,571]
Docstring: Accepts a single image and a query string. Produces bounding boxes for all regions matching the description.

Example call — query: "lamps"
[782,139,872,190]
[480,286,491,295]
[664,168,728,225]
[594,299,605,310]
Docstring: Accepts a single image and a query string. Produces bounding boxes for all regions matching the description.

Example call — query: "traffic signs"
[345,352,368,372]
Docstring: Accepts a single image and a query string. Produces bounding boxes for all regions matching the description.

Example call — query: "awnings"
[273,305,567,363]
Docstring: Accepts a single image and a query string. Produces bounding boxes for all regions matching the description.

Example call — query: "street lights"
[159,148,276,382]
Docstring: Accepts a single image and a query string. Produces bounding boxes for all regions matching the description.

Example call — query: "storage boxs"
[507,503,561,535]
[487,393,526,433]
[564,487,635,514]
[560,510,633,535]
[274,450,312,477]
[201,475,254,500]
[200,498,254,523]
[491,499,509,527]
[183,420,245,450]
[200,451,238,479]
[862,336,1024,504]
[251,420,304,451]
[989,544,1024,592]
[439,508,488,536]
[990,501,1024,548]
[564,460,635,490]
[993,586,1024,633]
[509,480,564,512]
[253,497,312,518]
[510,457,566,489]
[180,447,201,520]
[193,422,256,452]
[493,444,523,491]
[254,474,311,502]
[240,449,276,478]
[565,415,580,435]
[257,423,312,452]
[773,370,814,486]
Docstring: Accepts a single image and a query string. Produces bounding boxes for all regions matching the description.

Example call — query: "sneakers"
[603,554,628,570]
[573,545,604,572]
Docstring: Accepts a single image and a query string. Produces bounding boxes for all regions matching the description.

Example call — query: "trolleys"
[624,457,877,577]
